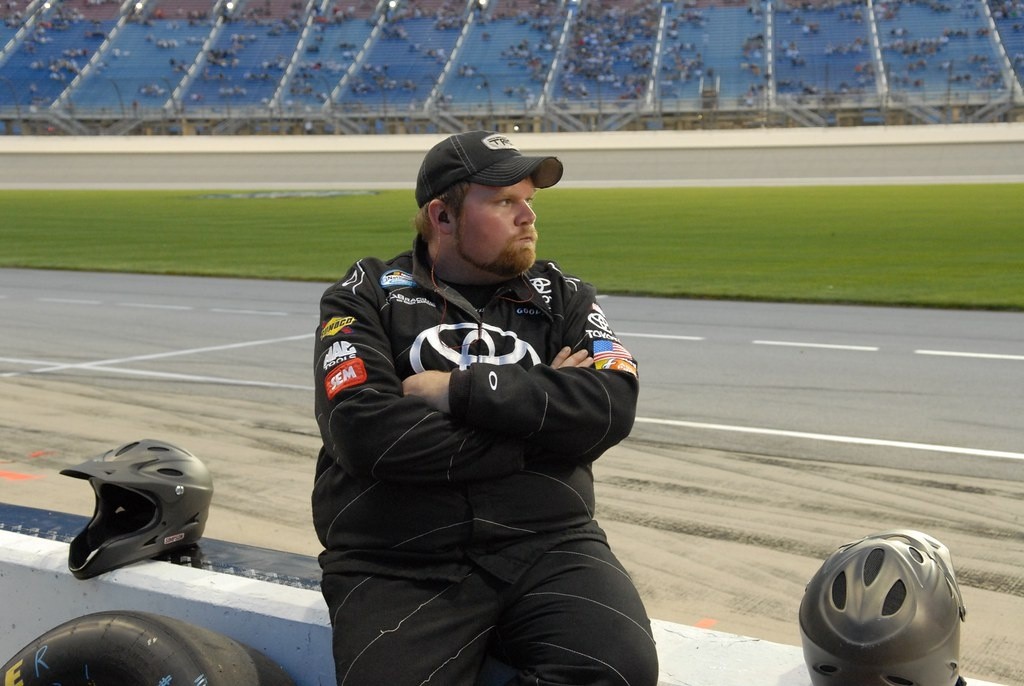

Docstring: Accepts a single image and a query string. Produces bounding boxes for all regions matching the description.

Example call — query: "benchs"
[0,0,1024,120]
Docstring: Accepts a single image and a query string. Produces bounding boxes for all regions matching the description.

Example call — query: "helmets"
[60,439,215,580]
[798,529,968,686]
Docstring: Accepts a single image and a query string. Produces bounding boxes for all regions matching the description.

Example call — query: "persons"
[312,130,661,686]
[0,0,1024,135]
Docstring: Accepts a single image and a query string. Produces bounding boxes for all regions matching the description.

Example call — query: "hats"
[415,130,564,209]
